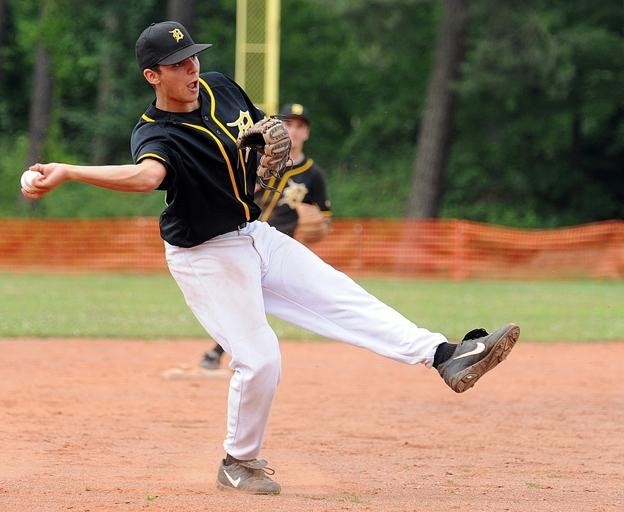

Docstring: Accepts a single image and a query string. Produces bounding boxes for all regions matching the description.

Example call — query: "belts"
[238,222,247,229]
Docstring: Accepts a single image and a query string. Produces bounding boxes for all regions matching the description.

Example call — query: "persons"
[198,104,331,370]
[21,21,520,494]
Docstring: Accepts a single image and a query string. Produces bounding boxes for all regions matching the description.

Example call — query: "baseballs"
[19,169,44,193]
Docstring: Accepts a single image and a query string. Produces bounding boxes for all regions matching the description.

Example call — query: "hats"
[135,21,213,71]
[270,103,311,126]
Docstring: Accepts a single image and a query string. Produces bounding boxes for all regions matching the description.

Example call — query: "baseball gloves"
[235,114,294,180]
[293,202,327,245]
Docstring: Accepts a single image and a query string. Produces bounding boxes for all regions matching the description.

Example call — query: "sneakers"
[215,458,281,496]
[196,352,221,369]
[437,323,520,394]
